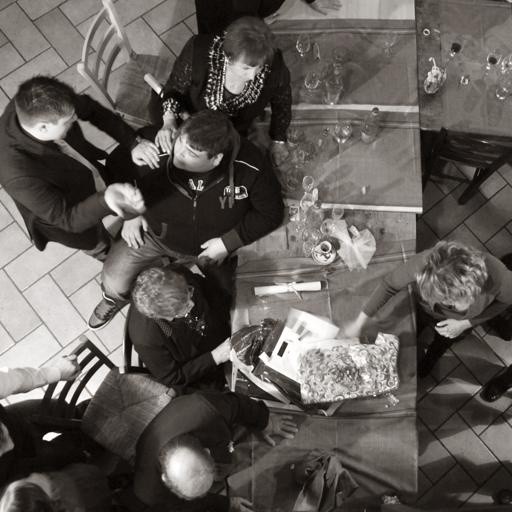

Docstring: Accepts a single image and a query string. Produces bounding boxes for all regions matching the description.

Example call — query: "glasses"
[176,285,195,317]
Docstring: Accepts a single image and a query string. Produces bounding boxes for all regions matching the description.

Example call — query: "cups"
[275,126,316,190]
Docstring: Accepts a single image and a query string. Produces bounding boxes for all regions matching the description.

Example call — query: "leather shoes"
[483,373,512,402]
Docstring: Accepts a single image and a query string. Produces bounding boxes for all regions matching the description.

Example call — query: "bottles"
[323,43,381,147]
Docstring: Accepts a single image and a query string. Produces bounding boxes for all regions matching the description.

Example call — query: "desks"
[227,0,425,512]
[414,1,511,137]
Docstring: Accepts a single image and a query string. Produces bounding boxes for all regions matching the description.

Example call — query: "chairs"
[422,127,511,205]
[40,337,176,478]
[78,0,176,124]
[123,293,151,374]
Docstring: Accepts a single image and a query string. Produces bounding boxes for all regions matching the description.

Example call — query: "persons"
[2,76,159,263]
[1,354,299,511]
[336,239,512,380]
[481,364,511,403]
[89,0,293,393]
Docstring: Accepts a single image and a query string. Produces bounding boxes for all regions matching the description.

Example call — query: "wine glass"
[304,71,319,92]
[484,48,512,101]
[296,31,311,59]
[445,42,464,61]
[292,177,345,267]
[379,28,399,57]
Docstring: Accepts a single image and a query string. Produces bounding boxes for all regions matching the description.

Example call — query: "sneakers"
[88,294,120,330]
